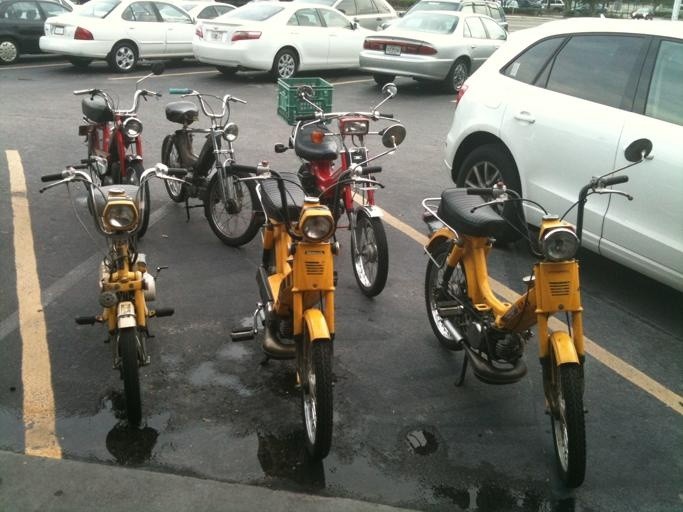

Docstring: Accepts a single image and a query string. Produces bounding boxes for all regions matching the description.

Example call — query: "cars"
[358,9,509,95]
[0,0,73,66]
[191,0,379,84]
[38,0,197,74]
[442,15,683,295]
[631,7,654,20]
[541,0,608,14]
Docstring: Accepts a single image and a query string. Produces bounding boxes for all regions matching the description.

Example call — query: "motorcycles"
[421,136,655,489]
[72,60,164,240]
[161,86,266,249]
[38,162,193,429]
[274,81,402,299]
[229,122,408,461]
